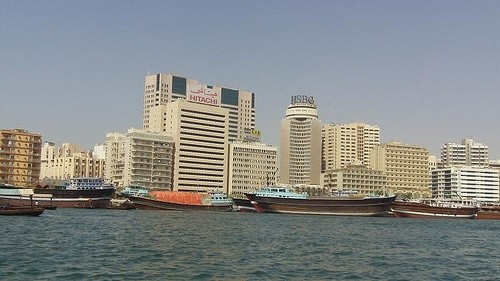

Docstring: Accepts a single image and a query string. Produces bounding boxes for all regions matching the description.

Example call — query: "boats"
[0,188,44,217]
[116,188,233,212]
[473,204,500,220]
[245,187,399,218]
[34,177,136,210]
[231,198,256,212]
[21,194,57,210]
[388,198,480,219]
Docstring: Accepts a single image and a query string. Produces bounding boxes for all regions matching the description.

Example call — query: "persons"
[5,203,10,210]
[35,200,38,209]
